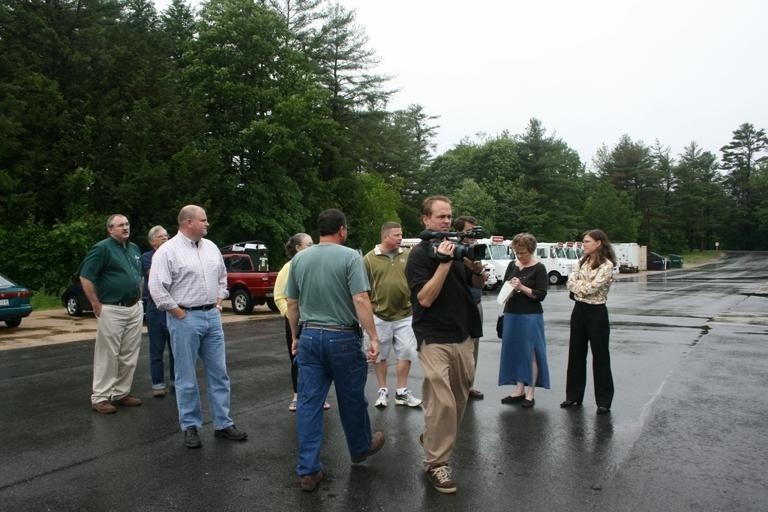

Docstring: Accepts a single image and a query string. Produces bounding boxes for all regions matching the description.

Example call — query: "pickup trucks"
[222,253,280,314]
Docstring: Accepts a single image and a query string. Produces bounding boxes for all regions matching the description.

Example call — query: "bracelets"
[215,303,222,310]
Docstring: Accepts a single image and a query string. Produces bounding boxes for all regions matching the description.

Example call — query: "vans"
[399,235,642,293]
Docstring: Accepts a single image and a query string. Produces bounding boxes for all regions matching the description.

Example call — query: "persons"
[286,209,384,492]
[148,206,248,449]
[362,222,423,409]
[453,216,490,402]
[276,232,329,412]
[79,214,144,414]
[498,233,551,409]
[407,196,483,493]
[560,229,617,414]
[141,226,176,398]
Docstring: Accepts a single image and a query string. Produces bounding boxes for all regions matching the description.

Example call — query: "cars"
[0,272,33,328]
[61,276,149,316]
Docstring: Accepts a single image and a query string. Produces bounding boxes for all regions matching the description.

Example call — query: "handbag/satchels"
[496,315,504,338]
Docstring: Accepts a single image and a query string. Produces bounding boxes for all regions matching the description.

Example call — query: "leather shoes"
[502,393,609,414]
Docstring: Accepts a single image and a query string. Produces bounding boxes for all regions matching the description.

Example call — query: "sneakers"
[92,389,247,448]
[289,387,484,493]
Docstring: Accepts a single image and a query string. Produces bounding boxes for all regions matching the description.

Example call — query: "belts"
[110,301,138,307]
[178,304,217,311]
